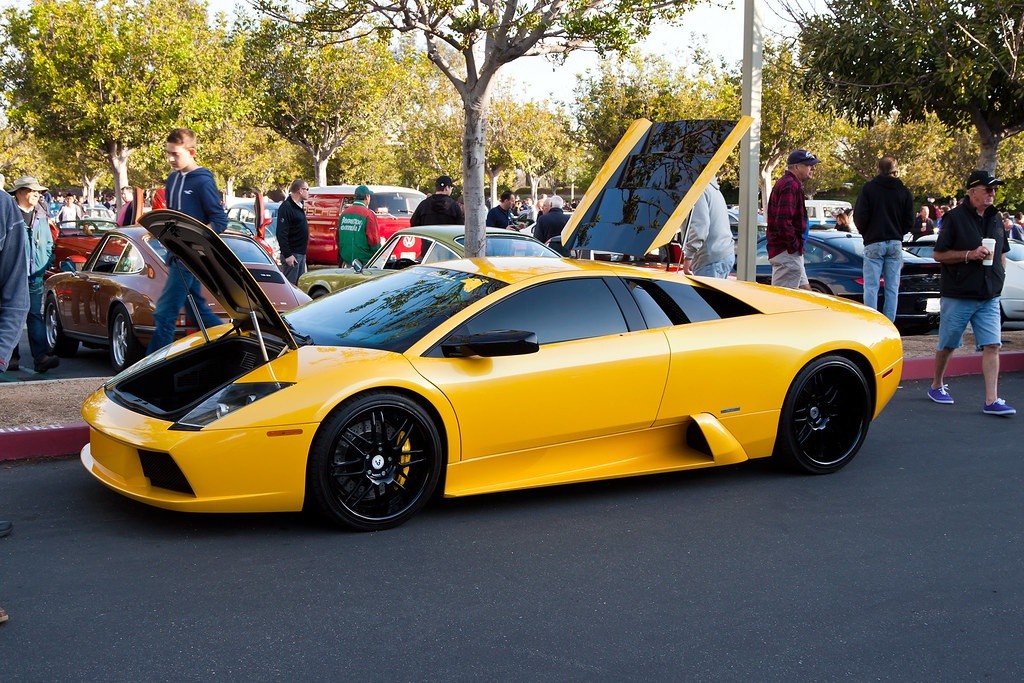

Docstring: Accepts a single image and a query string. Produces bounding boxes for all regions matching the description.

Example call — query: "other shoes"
[6,359,19,370]
[36,354,60,374]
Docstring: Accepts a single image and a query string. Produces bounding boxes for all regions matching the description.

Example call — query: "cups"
[921,222,926,231]
[981,238,996,266]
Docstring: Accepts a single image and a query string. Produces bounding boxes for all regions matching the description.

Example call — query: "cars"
[908,234,1024,327]
[730,228,943,329]
[227,202,282,238]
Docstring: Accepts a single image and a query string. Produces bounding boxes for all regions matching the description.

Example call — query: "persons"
[5,176,63,373]
[924,171,1017,414]
[1,189,32,374]
[852,156,917,323]
[766,149,822,292]
[835,196,1024,247]
[145,129,232,354]
[334,185,381,269]
[679,174,734,279]
[487,190,582,244]
[409,176,465,265]
[70,281,120,328]
[39,186,148,227]
[277,179,310,286]
[241,192,255,198]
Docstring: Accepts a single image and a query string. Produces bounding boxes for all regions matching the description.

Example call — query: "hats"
[7,177,49,194]
[355,185,373,197]
[966,170,1005,187]
[436,176,457,188]
[787,150,821,166]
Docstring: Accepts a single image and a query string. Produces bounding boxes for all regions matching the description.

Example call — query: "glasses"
[24,188,39,192]
[969,187,998,193]
[298,188,308,191]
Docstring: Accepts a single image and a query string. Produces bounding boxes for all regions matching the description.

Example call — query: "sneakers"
[982,398,1016,415]
[926,384,954,404]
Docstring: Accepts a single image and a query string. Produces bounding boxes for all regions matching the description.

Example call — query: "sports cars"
[47,218,129,272]
[296,225,564,302]
[80,116,903,527]
[46,225,314,367]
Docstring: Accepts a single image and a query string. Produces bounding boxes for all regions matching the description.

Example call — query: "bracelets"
[965,250,972,265]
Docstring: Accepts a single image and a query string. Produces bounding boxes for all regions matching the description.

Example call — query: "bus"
[302,185,426,262]
